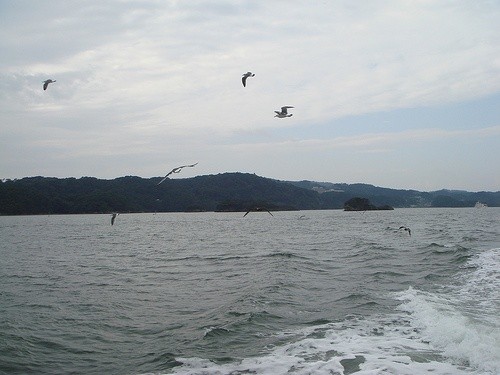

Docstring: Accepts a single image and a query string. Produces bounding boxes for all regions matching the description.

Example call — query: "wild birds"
[42,79,57,90]
[111,212,120,225]
[157,162,199,185]
[243,208,274,217]
[241,72,255,87]
[274,106,294,118]
[398,226,412,237]
[358,209,369,216]
[294,215,306,220]
